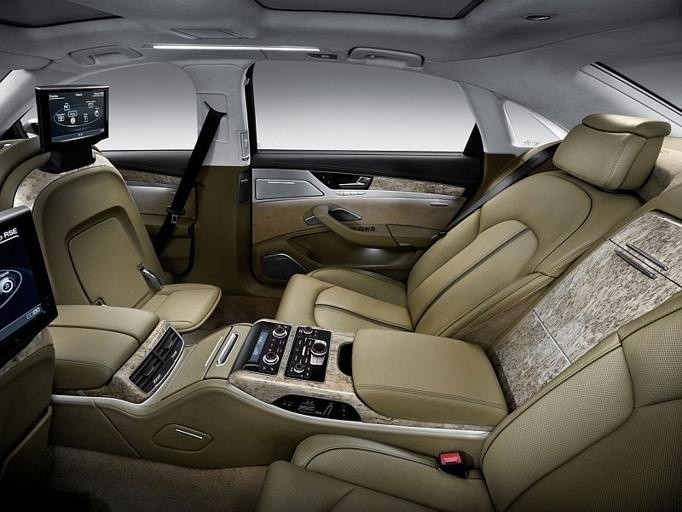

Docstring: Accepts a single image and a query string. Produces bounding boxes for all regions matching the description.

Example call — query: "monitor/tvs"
[35,83,109,151]
[0,206,58,369]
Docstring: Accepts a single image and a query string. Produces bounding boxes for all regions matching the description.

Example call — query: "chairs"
[247,289,682,510]
[270,110,672,343]
[36,173,224,331]
